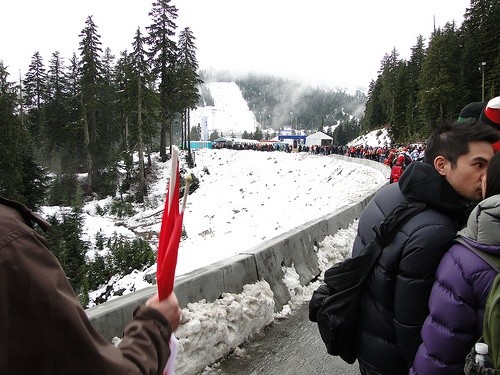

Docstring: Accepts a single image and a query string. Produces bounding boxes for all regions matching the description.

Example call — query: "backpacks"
[454,235,500,375]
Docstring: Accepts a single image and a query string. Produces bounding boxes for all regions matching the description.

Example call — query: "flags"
[156,145,190,375]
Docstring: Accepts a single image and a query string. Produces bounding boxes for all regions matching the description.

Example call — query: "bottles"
[474,343,495,370]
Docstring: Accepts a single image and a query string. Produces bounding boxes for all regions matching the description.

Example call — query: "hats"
[479,95,500,130]
[458,102,486,124]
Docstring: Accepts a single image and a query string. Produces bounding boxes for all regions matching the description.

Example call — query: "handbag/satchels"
[308,201,433,364]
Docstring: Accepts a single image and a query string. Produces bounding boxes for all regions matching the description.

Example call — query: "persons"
[0,196,182,375]
[213,96,500,184]
[308,96,500,375]
